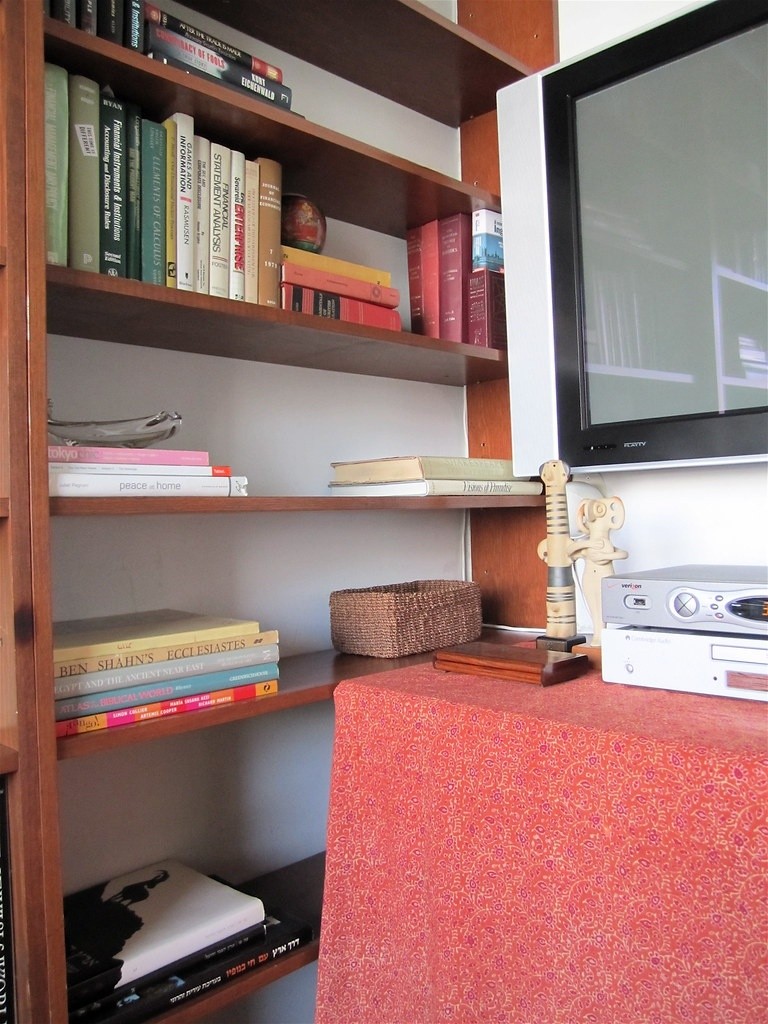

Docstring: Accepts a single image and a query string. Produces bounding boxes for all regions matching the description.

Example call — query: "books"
[46,0,506,351]
[328,455,543,496]
[48,445,248,497]
[63,857,315,1024]
[584,201,767,381]
[53,607,280,738]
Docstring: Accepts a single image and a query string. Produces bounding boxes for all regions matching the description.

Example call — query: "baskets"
[329,578,482,659]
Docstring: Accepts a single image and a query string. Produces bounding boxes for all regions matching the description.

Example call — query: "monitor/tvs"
[497,0,768,476]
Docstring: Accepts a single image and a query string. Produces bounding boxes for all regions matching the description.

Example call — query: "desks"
[311,640,768,1024]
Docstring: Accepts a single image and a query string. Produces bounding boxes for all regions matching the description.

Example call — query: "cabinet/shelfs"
[0,0,582,1024]
